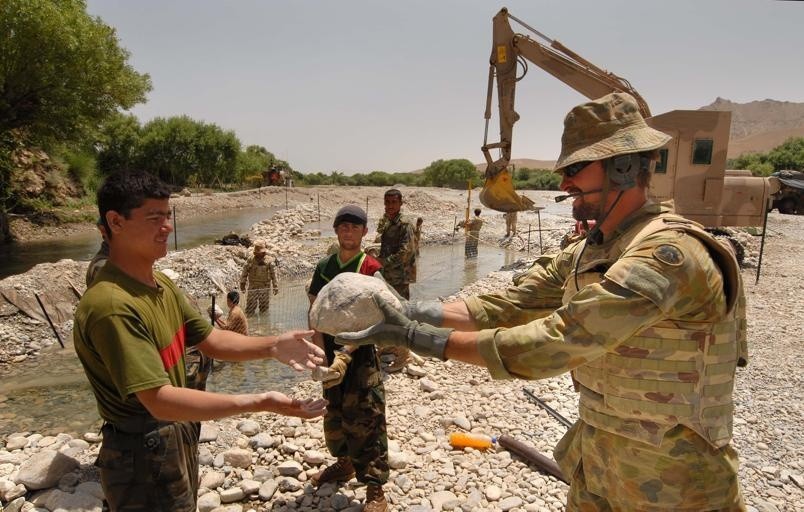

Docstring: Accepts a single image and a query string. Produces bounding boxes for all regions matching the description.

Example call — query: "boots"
[310,453,357,483]
[362,479,389,512]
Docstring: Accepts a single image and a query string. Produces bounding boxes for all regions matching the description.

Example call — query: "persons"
[85,218,109,288]
[377,206,392,234]
[415,218,423,255]
[308,205,388,512]
[209,292,248,336]
[376,190,417,373]
[240,239,279,315]
[466,209,482,259]
[74,169,330,512]
[504,211,516,237]
[333,93,750,512]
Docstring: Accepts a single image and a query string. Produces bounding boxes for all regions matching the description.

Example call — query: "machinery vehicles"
[260,157,294,187]
[479,7,781,286]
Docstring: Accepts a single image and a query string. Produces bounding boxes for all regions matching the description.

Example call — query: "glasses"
[558,158,599,180]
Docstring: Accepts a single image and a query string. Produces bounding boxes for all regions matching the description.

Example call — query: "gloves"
[331,291,456,365]
[323,349,353,390]
[383,276,450,330]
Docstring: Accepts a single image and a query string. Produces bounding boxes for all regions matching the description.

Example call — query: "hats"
[332,204,367,227]
[548,91,674,176]
[253,240,268,254]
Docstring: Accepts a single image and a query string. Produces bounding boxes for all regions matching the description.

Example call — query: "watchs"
[338,346,351,353]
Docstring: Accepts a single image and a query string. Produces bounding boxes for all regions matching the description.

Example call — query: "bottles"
[451,433,497,449]
